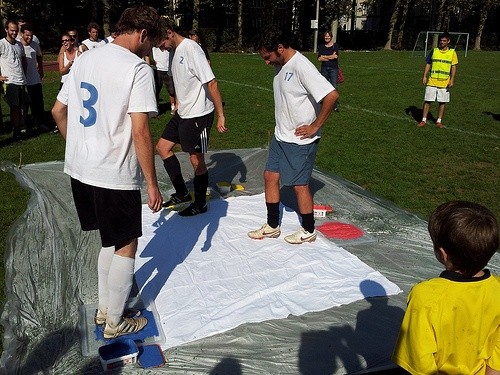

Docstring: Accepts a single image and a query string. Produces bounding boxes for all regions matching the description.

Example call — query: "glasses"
[61,39,72,43]
[69,35,77,38]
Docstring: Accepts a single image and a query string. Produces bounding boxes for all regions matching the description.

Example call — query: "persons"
[417,32,458,128]
[57,19,211,116]
[147,18,228,217]
[396,200,500,375]
[0,20,44,132]
[50,6,165,339]
[248,29,339,244]
[317,30,338,112]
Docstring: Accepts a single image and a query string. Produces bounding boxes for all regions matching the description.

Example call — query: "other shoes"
[13,133,23,141]
[435,122,442,128]
[417,121,426,126]
[333,107,338,112]
[35,122,51,131]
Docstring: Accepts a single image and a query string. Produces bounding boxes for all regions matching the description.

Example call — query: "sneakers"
[94,309,147,340]
[248,223,280,239]
[162,192,192,208]
[284,226,316,244]
[178,202,207,216]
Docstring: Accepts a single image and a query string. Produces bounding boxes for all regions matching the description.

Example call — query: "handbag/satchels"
[338,67,344,84]
